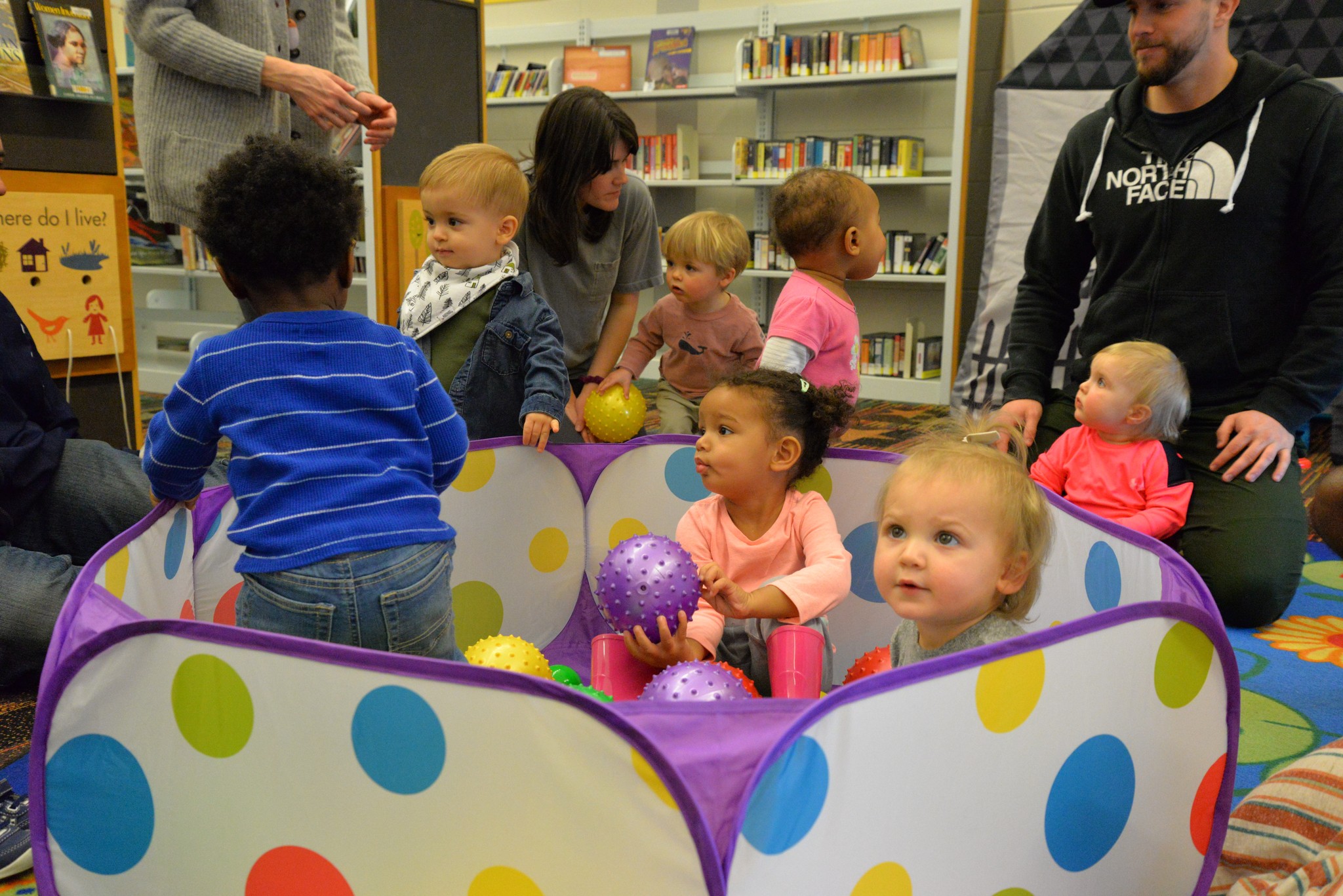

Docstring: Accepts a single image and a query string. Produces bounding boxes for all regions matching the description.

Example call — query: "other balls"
[842,645,893,684]
[583,383,647,444]
[464,633,555,685]
[567,683,613,703]
[594,531,702,644]
[639,659,763,702]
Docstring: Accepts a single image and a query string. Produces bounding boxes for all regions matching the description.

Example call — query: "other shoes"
[0,776,36,878]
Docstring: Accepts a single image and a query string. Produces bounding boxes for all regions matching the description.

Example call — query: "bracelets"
[580,374,605,387]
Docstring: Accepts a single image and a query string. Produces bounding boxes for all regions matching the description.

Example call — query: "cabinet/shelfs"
[483,0,1009,407]
[110,0,485,394]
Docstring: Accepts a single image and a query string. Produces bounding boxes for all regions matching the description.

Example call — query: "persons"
[623,367,1057,696]
[511,85,664,444]
[598,166,887,435]
[0,290,153,689]
[397,142,571,451]
[1000,0,1343,629]
[123,0,396,233]
[46,19,100,92]
[140,128,470,661]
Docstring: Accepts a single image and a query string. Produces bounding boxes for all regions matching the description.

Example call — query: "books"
[625,126,699,181]
[743,24,927,78]
[26,0,114,104]
[119,76,182,267]
[0,0,33,94]
[643,26,696,91]
[196,195,366,275]
[561,45,632,92]
[736,133,948,378]
[486,62,548,97]
